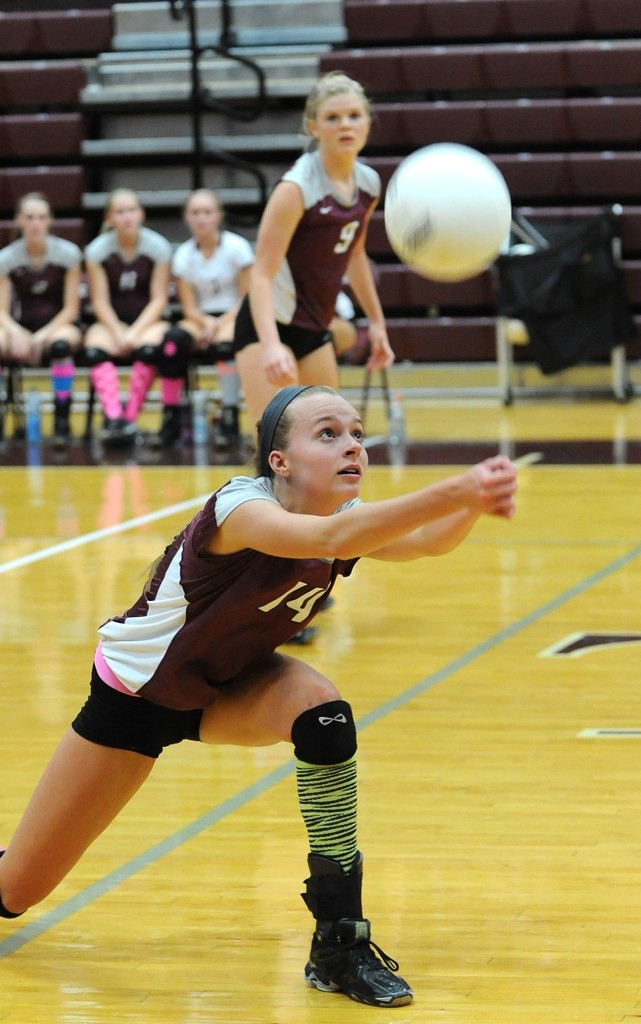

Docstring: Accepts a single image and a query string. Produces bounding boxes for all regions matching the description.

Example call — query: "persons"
[0,191,83,452]
[0,382,520,1008]
[151,187,257,454]
[84,185,173,450]
[247,69,395,647]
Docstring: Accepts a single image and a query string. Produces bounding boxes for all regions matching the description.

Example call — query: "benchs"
[0,0,641,457]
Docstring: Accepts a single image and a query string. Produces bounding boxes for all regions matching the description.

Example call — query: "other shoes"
[215,405,240,447]
[150,408,183,449]
[99,418,135,450]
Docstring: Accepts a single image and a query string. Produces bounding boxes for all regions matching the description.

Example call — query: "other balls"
[384,142,511,283]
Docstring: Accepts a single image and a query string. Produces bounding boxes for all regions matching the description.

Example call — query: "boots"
[53,399,73,449]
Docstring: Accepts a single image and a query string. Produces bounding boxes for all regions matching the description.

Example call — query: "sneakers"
[305,918,414,1008]
